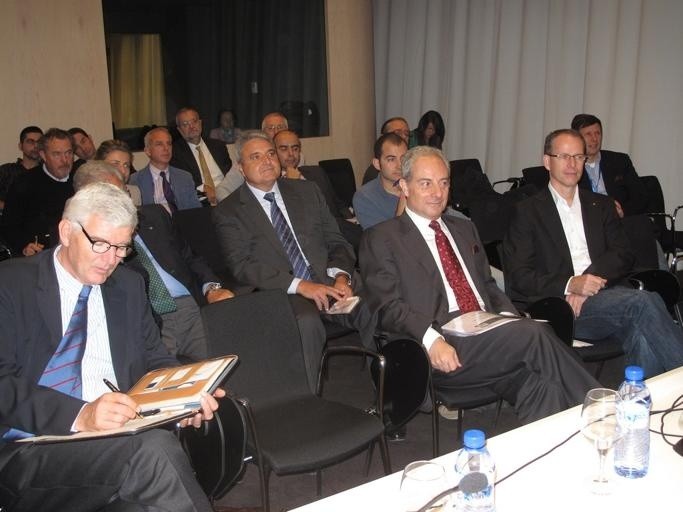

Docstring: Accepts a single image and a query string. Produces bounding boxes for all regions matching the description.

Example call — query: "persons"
[72,158,234,368]
[0,180,226,512]
[359,145,605,426]
[501,130,683,380]
[127,127,202,222]
[361,117,410,185]
[168,107,232,207]
[413,110,445,151]
[571,113,658,271]
[352,132,410,231]
[0,118,44,230]
[214,112,305,205]
[0,127,76,257]
[95,139,133,184]
[67,127,96,164]
[273,129,364,256]
[208,108,243,144]
[212,129,406,439]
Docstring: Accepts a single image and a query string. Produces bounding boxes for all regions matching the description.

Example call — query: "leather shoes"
[383,414,407,443]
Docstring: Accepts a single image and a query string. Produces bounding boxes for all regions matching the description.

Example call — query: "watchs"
[205,284,222,299]
[335,274,350,286]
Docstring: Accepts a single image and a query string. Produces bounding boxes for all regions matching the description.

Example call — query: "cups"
[580,387,621,487]
[402,459,448,512]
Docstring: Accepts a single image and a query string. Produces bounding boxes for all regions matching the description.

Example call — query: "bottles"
[455,430,496,512]
[613,367,651,479]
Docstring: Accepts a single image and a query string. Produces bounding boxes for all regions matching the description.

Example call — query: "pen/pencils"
[104,379,146,420]
[35,235,38,253]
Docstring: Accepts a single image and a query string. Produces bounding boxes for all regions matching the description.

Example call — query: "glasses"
[78,220,133,259]
[549,153,588,163]
[266,125,285,131]
[179,120,197,127]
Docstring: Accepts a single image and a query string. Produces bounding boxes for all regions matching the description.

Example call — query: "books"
[441,310,548,337]
[328,296,361,314]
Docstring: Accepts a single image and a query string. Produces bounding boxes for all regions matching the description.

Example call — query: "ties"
[3,284,92,438]
[131,240,177,315]
[429,220,481,315]
[160,171,178,214]
[196,146,218,206]
[263,192,313,283]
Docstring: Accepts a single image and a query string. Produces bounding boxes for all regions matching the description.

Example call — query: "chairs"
[319,158,357,208]
[199,287,393,512]
[634,174,683,255]
[171,207,214,234]
[447,158,518,251]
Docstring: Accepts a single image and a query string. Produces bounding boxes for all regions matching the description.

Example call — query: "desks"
[286,363,683,511]
